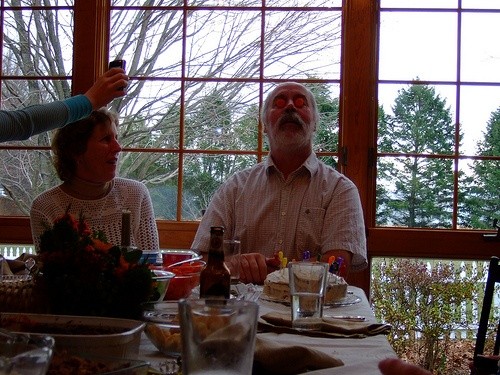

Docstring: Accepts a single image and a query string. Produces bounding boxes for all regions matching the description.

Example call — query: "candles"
[122,208,131,246]
[279,250,346,282]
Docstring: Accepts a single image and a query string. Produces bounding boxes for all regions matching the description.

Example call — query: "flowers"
[28,202,161,320]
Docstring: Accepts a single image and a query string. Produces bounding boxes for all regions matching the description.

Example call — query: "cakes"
[263,262,348,304]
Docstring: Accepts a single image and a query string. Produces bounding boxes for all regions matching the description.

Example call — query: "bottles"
[199,227,230,299]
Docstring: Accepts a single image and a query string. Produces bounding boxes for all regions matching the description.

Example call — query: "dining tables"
[137,283,399,375]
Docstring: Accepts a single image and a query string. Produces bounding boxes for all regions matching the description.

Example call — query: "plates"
[260,292,363,306]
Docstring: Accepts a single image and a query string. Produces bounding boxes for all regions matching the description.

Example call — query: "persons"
[30,106,159,256]
[185,82,369,284]
[0,67,131,143]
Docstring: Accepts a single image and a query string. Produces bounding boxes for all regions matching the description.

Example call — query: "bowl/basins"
[137,300,183,357]
[37,270,176,321]
[0,332,55,375]
[0,313,145,361]
[165,273,193,301]
[163,262,206,289]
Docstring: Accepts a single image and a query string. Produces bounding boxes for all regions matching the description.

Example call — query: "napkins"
[200,322,344,375]
[258,311,391,339]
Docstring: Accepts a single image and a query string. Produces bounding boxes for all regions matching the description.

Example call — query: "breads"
[143,305,232,352]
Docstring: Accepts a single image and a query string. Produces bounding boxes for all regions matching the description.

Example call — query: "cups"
[223,239,242,294]
[178,298,259,375]
[288,261,329,330]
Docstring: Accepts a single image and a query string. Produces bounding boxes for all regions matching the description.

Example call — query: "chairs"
[472,256,500,375]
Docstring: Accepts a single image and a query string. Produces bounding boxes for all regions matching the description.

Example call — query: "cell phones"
[109,60,126,91]
